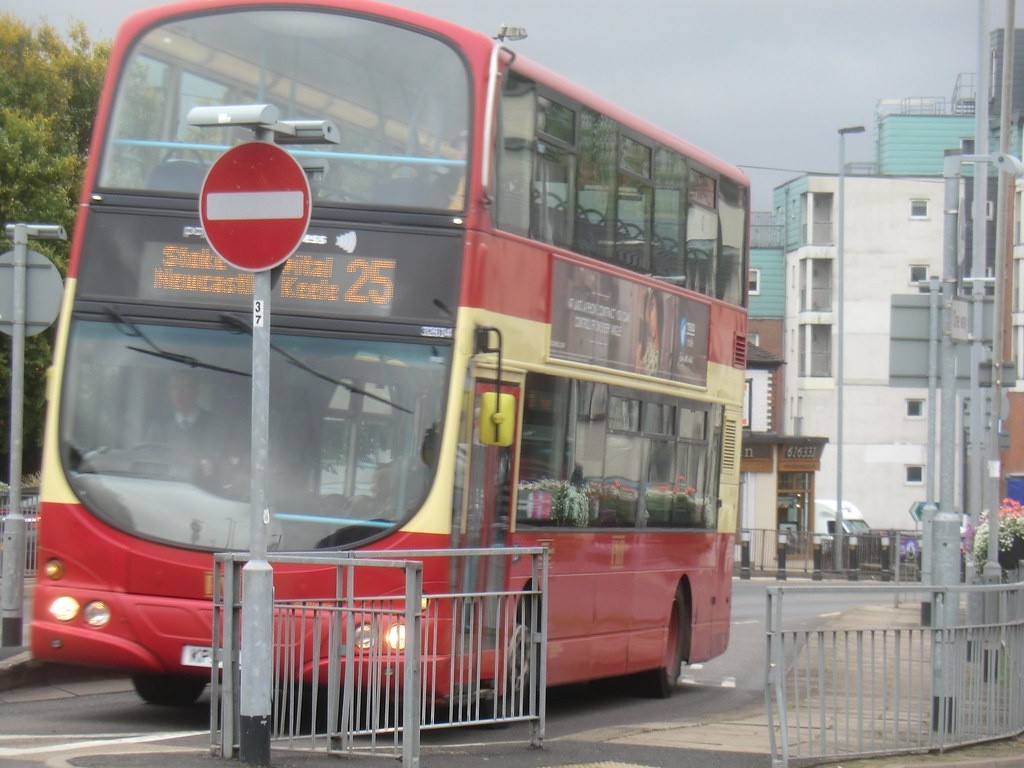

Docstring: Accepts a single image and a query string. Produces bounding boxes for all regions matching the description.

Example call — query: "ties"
[182,420,188,431]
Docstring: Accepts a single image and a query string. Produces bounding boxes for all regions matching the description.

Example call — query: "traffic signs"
[910,501,940,523]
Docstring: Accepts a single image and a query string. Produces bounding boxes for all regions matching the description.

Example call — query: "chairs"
[147,146,733,302]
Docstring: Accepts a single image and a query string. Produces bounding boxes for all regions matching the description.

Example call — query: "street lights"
[185,103,342,766]
[0,224,68,647]
[831,125,867,580]
[931,151,1024,735]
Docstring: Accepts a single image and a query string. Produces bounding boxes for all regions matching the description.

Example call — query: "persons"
[783,526,799,553]
[626,288,668,373]
[151,370,230,493]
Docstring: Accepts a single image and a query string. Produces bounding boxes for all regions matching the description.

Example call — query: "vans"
[777,499,874,550]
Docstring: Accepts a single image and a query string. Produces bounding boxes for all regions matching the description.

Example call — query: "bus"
[32,2,751,732]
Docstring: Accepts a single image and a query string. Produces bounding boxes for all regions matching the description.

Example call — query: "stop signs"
[197,141,312,273]
